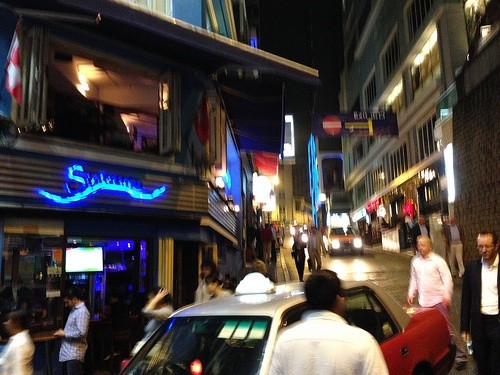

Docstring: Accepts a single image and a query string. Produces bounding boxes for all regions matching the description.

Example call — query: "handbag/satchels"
[292,251,295,256]
[308,258,312,271]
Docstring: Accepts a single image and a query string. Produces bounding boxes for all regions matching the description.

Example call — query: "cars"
[119,273,458,375]
[323,226,364,256]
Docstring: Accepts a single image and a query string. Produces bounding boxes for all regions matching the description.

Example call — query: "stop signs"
[322,115,343,135]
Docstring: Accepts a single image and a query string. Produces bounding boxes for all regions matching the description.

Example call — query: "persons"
[129,285,177,375]
[460,230,500,375]
[53,285,90,375]
[269,269,390,375]
[292,233,307,282]
[194,259,267,302]
[0,310,35,375]
[407,214,434,255]
[407,236,469,370]
[441,218,466,278]
[256,223,280,266]
[307,227,326,274]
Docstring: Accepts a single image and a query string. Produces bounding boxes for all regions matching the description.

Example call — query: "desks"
[0,330,58,375]
